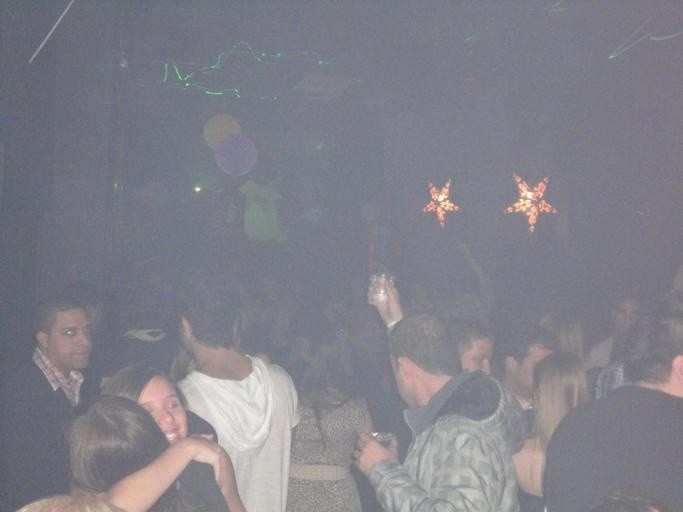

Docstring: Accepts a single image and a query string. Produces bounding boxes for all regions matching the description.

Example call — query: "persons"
[1,268,683,512]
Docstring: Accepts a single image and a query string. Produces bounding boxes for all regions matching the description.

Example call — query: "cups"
[368,430,396,446]
[365,271,396,309]
